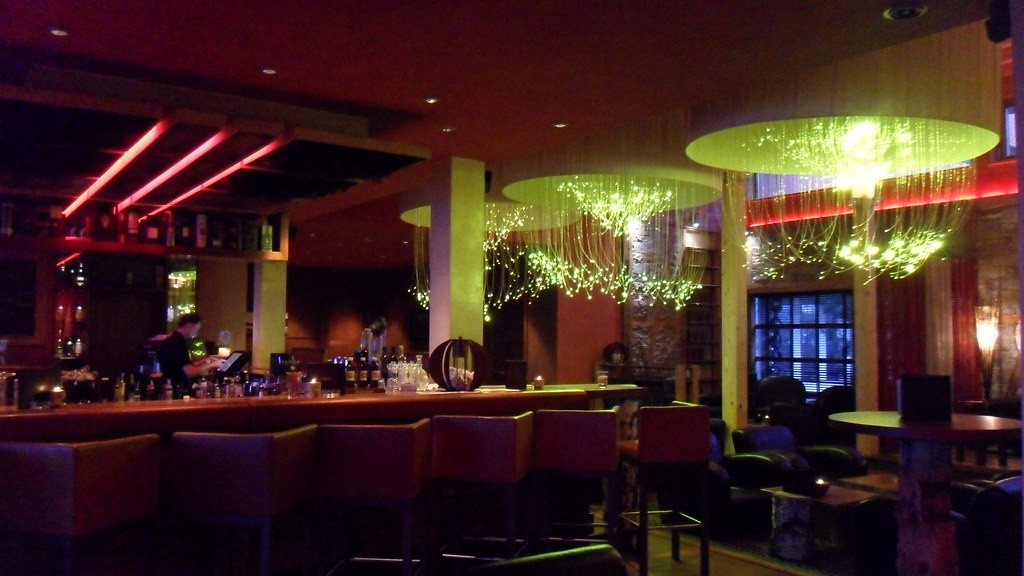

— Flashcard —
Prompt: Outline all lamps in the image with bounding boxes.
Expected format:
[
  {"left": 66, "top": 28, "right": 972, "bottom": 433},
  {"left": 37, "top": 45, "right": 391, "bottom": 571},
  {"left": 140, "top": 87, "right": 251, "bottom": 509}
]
[{"left": 401, "top": 20, "right": 1003, "bottom": 324}]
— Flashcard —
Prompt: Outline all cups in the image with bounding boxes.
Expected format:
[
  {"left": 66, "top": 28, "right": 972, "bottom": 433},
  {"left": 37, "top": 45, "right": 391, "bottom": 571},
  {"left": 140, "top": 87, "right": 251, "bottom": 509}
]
[{"left": 596, "top": 370, "right": 608, "bottom": 388}]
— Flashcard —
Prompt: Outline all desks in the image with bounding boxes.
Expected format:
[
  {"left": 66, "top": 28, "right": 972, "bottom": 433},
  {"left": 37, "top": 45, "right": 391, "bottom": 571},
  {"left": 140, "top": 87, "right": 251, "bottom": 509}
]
[
  {"left": 760, "top": 474, "right": 903, "bottom": 568},
  {"left": 830, "top": 411, "right": 1023, "bottom": 576}
]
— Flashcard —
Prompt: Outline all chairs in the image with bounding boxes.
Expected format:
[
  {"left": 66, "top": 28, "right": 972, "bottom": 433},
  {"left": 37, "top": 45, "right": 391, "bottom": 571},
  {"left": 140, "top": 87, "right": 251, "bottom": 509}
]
[{"left": 661, "top": 374, "right": 1023, "bottom": 576}]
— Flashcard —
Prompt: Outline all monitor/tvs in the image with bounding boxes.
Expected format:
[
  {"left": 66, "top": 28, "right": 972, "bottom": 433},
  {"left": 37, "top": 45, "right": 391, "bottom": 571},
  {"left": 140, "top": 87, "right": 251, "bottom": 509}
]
[{"left": 216, "top": 351, "right": 250, "bottom": 377}]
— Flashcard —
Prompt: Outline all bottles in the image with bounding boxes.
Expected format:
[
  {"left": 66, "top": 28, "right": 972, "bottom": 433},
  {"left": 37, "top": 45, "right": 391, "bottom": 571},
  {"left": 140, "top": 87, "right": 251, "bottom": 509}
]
[
  {"left": 146, "top": 214, "right": 161, "bottom": 243},
  {"left": 212, "top": 222, "right": 223, "bottom": 249},
  {"left": 96, "top": 368, "right": 284, "bottom": 403},
  {"left": 178, "top": 211, "right": 193, "bottom": 246},
  {"left": 344, "top": 344, "right": 406, "bottom": 391}
]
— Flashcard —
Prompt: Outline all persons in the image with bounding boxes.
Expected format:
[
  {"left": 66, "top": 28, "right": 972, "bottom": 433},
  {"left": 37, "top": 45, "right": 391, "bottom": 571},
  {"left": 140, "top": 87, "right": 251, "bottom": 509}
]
[
  {"left": 63, "top": 321, "right": 89, "bottom": 369},
  {"left": 158, "top": 314, "right": 227, "bottom": 389}
]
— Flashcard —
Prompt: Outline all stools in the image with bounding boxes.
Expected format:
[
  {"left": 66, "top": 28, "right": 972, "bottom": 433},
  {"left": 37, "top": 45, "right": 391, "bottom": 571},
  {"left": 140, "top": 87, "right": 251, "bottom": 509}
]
[{"left": 0, "top": 401, "right": 711, "bottom": 575}]
